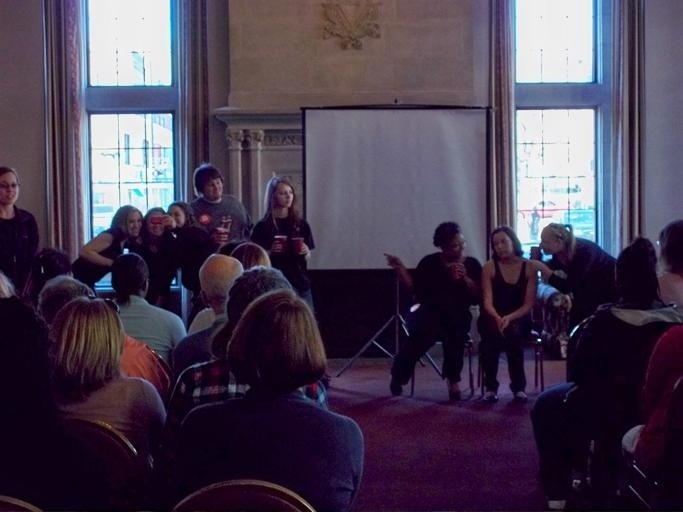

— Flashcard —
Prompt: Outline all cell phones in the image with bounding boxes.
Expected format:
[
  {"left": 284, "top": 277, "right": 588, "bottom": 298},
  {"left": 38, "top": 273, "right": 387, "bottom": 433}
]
[
  {"left": 531, "top": 247, "right": 539, "bottom": 259},
  {"left": 151, "top": 215, "right": 168, "bottom": 224}
]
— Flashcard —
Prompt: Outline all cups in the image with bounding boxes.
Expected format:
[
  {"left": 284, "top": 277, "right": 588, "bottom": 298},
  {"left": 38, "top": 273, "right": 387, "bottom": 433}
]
[
  {"left": 274, "top": 236, "right": 288, "bottom": 253},
  {"left": 216, "top": 229, "right": 230, "bottom": 243},
  {"left": 291, "top": 237, "right": 304, "bottom": 255}
]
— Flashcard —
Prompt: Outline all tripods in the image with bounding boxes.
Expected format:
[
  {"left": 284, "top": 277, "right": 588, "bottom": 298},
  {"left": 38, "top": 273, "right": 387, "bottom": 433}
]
[{"left": 336, "top": 277, "right": 444, "bottom": 396}]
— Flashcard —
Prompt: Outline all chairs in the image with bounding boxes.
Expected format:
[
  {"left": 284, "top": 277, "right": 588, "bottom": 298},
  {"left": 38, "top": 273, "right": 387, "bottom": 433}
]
[
  {"left": 563, "top": 314, "right": 595, "bottom": 385},
  {"left": 149, "top": 344, "right": 178, "bottom": 390},
  {"left": 50, "top": 412, "right": 158, "bottom": 512},
  {"left": 403, "top": 286, "right": 482, "bottom": 400},
  {"left": 168, "top": 477, "right": 320, "bottom": 512},
  {"left": 0, "top": 493, "right": 45, "bottom": 511},
  {"left": 618, "top": 371, "right": 683, "bottom": 512},
  {"left": 477, "top": 284, "right": 548, "bottom": 400}
]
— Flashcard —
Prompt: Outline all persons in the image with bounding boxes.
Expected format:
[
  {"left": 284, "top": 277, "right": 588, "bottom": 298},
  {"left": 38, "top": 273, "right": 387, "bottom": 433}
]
[
  {"left": 620, "top": 220, "right": 683, "bottom": 511},
  {"left": 481, "top": 227, "right": 539, "bottom": 403},
  {"left": 1, "top": 241, "right": 366, "bottom": 512},
  {"left": 525, "top": 223, "right": 618, "bottom": 335},
  {"left": 384, "top": 222, "right": 483, "bottom": 402},
  {"left": 1, "top": 168, "right": 40, "bottom": 297},
  {"left": 530, "top": 237, "right": 683, "bottom": 511},
  {"left": 70, "top": 164, "right": 318, "bottom": 325}
]
